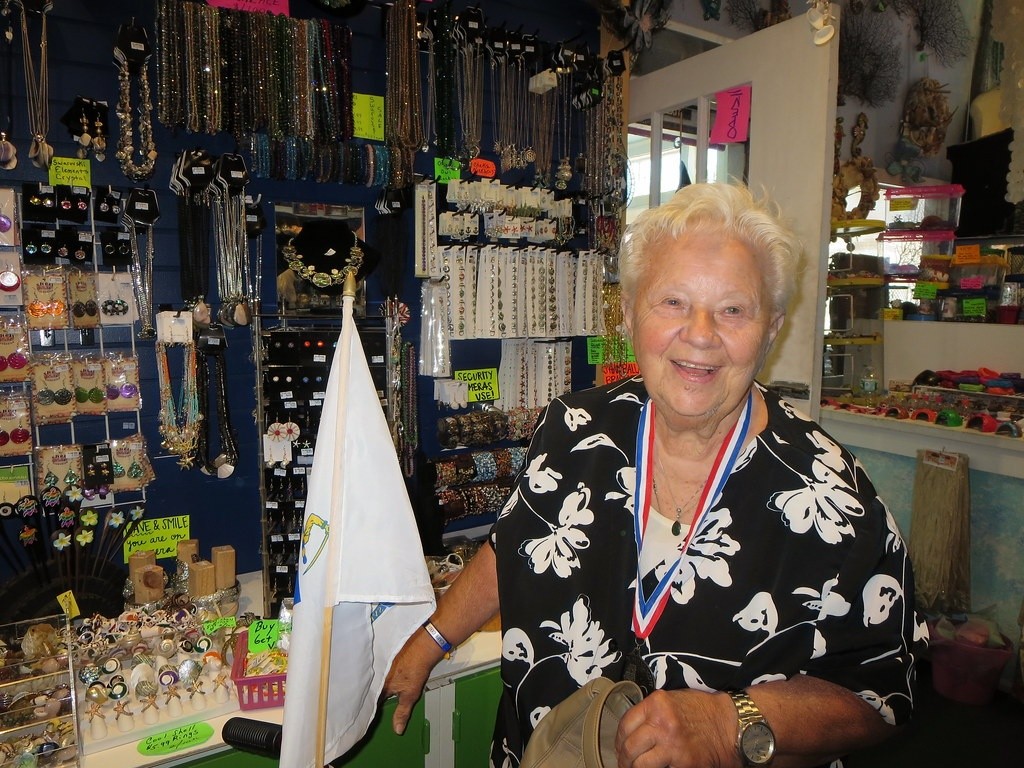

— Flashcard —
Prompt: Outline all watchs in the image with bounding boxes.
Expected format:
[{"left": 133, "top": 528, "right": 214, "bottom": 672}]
[{"left": 726, "top": 690, "right": 776, "bottom": 768}]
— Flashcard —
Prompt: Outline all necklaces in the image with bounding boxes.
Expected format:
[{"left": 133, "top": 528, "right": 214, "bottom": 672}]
[
  {"left": 384, "top": 0, "right": 605, "bottom": 199},
  {"left": 651, "top": 478, "right": 662, "bottom": 515},
  {"left": 654, "top": 440, "right": 708, "bottom": 536},
  {"left": 0, "top": 0, "right": 353, "bottom": 480}
]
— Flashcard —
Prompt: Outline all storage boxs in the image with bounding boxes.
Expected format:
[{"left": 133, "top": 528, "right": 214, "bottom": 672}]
[
  {"left": 925, "top": 618, "right": 1014, "bottom": 706},
  {"left": 230, "top": 628, "right": 287, "bottom": 712}
]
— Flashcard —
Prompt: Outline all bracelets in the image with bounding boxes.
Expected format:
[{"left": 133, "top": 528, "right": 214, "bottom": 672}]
[
  {"left": 418, "top": 192, "right": 630, "bottom": 523},
  {"left": 422, "top": 620, "right": 451, "bottom": 660}
]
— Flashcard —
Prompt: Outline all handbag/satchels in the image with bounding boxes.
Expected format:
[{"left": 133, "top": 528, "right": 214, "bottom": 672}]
[{"left": 519, "top": 676, "right": 644, "bottom": 768}]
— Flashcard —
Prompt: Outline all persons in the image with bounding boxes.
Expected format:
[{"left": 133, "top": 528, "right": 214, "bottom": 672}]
[{"left": 382, "top": 186, "right": 930, "bottom": 768}]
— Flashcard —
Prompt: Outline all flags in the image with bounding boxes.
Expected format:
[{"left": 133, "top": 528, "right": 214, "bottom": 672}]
[{"left": 279, "top": 320, "right": 437, "bottom": 768}]
